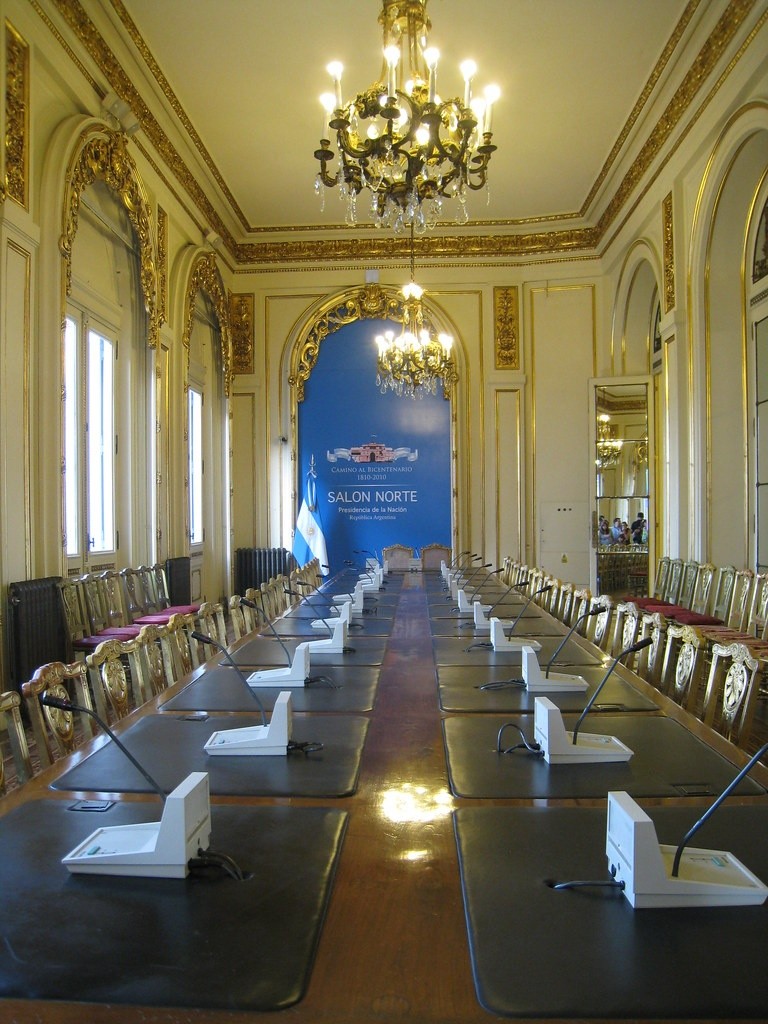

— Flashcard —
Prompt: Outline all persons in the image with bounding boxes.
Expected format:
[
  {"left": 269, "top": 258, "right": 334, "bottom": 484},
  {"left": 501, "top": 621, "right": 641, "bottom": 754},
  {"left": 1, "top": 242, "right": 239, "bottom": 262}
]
[{"left": 598, "top": 512, "right": 648, "bottom": 545}]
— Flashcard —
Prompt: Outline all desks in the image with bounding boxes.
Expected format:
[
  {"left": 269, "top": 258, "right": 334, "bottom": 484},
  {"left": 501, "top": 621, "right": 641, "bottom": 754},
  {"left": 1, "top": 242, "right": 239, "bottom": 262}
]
[{"left": 0, "top": 569, "right": 768, "bottom": 1024}]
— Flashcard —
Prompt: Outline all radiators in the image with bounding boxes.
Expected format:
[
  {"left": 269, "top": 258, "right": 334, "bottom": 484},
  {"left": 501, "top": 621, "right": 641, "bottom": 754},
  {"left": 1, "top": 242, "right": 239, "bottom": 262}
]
[
  {"left": 8, "top": 576, "right": 77, "bottom": 729},
  {"left": 165, "top": 557, "right": 192, "bottom": 607},
  {"left": 234, "top": 547, "right": 292, "bottom": 598}
]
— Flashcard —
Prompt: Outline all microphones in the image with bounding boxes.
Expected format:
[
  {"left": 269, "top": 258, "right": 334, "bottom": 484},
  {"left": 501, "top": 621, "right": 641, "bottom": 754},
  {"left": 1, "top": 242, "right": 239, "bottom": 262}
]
[
  {"left": 42, "top": 695, "right": 212, "bottom": 879},
  {"left": 189, "top": 550, "right": 384, "bottom": 755},
  {"left": 441, "top": 550, "right": 653, "bottom": 764}
]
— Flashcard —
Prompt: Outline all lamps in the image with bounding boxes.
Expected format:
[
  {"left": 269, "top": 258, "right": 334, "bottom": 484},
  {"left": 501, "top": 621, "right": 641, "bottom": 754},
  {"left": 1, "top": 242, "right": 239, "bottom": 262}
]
[
  {"left": 374, "top": 225, "right": 460, "bottom": 403},
  {"left": 314, "top": 0, "right": 504, "bottom": 235}
]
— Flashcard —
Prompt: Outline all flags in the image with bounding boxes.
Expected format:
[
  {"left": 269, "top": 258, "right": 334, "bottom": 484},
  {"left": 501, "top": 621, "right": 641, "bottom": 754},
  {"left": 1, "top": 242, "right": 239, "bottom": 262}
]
[{"left": 291, "top": 471, "right": 330, "bottom": 577}]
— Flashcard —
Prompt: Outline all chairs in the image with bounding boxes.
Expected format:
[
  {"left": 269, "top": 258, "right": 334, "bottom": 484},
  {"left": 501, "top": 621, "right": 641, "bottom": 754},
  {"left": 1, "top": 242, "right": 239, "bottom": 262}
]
[
  {"left": 500, "top": 556, "right": 763, "bottom": 747},
  {"left": 0, "top": 557, "right": 325, "bottom": 799},
  {"left": 382, "top": 544, "right": 414, "bottom": 568},
  {"left": 420, "top": 544, "right": 452, "bottom": 569},
  {"left": 598, "top": 542, "right": 768, "bottom": 662},
  {"left": 597, "top": 543, "right": 648, "bottom": 598}
]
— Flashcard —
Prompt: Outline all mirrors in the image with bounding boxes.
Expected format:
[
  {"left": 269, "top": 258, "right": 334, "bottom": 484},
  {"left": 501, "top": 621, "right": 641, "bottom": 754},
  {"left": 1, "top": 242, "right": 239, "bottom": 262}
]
[{"left": 588, "top": 376, "right": 655, "bottom": 597}]
